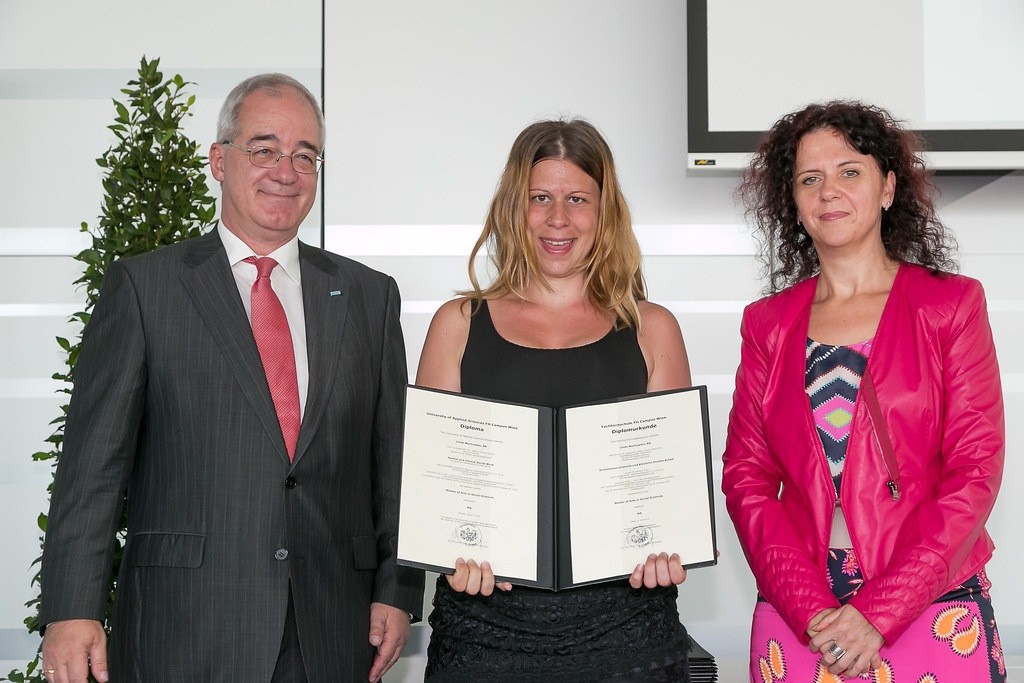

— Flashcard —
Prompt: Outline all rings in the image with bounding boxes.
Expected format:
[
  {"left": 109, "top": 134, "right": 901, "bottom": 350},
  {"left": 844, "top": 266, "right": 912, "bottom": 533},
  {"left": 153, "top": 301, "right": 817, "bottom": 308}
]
[
  {"left": 45, "top": 669, "right": 54, "bottom": 674},
  {"left": 829, "top": 644, "right": 846, "bottom": 659}
]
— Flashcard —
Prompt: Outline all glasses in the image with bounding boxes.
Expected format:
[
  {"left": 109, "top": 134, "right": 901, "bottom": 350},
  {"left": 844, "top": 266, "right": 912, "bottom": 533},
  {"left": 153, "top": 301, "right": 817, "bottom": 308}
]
[{"left": 229, "top": 141, "right": 326, "bottom": 175}]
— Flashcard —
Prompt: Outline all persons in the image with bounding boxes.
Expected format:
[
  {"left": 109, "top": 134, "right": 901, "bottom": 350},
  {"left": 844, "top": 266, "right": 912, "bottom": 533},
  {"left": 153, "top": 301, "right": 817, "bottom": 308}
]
[
  {"left": 39, "top": 75, "right": 426, "bottom": 683},
  {"left": 414, "top": 119, "right": 693, "bottom": 683},
  {"left": 720, "top": 101, "right": 1005, "bottom": 683}
]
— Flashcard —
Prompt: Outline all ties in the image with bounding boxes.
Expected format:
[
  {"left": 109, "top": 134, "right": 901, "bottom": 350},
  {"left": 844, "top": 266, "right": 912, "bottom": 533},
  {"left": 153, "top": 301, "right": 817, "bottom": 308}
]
[{"left": 239, "top": 255, "right": 301, "bottom": 464}]
